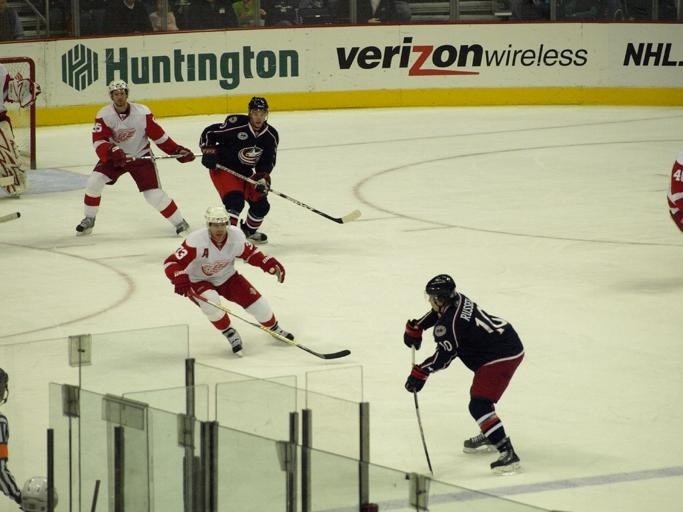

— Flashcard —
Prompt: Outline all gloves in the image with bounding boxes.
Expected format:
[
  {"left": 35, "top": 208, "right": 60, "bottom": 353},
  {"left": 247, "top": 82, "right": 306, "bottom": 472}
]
[
  {"left": 172, "top": 272, "right": 194, "bottom": 300},
  {"left": 200, "top": 149, "right": 224, "bottom": 169},
  {"left": 252, "top": 172, "right": 271, "bottom": 196},
  {"left": 261, "top": 254, "right": 285, "bottom": 282},
  {"left": 403, "top": 363, "right": 431, "bottom": 392},
  {"left": 172, "top": 145, "right": 196, "bottom": 163},
  {"left": 401, "top": 318, "right": 424, "bottom": 351},
  {"left": 97, "top": 143, "right": 127, "bottom": 173}
]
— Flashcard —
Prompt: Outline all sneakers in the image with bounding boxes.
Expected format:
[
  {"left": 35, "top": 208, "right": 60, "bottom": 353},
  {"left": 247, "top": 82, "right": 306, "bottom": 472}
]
[
  {"left": 74, "top": 215, "right": 96, "bottom": 234},
  {"left": 173, "top": 218, "right": 191, "bottom": 234}
]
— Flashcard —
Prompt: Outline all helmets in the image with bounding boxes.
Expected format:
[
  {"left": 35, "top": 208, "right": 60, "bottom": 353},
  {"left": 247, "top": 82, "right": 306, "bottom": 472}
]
[
  {"left": 0, "top": 367, "right": 8, "bottom": 409},
  {"left": 248, "top": 95, "right": 269, "bottom": 123},
  {"left": 107, "top": 79, "right": 128, "bottom": 97},
  {"left": 204, "top": 204, "right": 232, "bottom": 229},
  {"left": 423, "top": 271, "right": 457, "bottom": 308}
]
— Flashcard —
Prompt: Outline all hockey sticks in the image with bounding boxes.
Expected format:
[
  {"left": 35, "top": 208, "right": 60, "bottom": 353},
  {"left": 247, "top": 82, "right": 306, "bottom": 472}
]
[
  {"left": 191, "top": 292, "right": 351, "bottom": 359},
  {"left": 405, "top": 343, "right": 437, "bottom": 480},
  {"left": 216, "top": 165, "right": 362, "bottom": 223}
]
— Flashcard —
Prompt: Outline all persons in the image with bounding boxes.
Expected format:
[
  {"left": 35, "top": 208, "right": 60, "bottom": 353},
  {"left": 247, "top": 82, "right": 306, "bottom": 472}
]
[
  {"left": 404, "top": 274, "right": 525, "bottom": 469},
  {"left": 75, "top": 79, "right": 195, "bottom": 234},
  {"left": 666, "top": 154, "right": 683, "bottom": 231},
  {"left": 199, "top": 96, "right": 279, "bottom": 240},
  {"left": 0, "top": 367, "right": 23, "bottom": 504},
  {"left": 163, "top": 204, "right": 294, "bottom": 352},
  {"left": 508, "top": 1, "right": 678, "bottom": 19}
]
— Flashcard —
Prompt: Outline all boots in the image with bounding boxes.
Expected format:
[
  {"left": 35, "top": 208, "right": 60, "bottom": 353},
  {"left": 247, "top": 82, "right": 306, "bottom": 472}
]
[
  {"left": 221, "top": 325, "right": 243, "bottom": 355},
  {"left": 489, "top": 436, "right": 522, "bottom": 470},
  {"left": 268, "top": 322, "right": 295, "bottom": 342},
  {"left": 463, "top": 429, "right": 496, "bottom": 448},
  {"left": 240, "top": 222, "right": 267, "bottom": 242}
]
